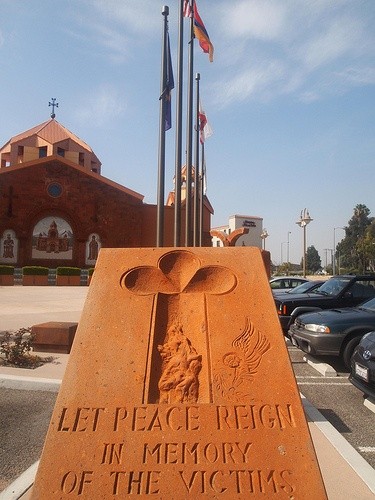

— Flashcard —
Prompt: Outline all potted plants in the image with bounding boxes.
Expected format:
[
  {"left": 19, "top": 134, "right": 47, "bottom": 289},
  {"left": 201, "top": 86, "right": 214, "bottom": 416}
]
[
  {"left": 0, "top": 266, "right": 14, "bottom": 286},
  {"left": 22, "top": 266, "right": 49, "bottom": 286},
  {"left": 55, "top": 266, "right": 81, "bottom": 285},
  {"left": 87, "top": 268, "right": 94, "bottom": 286}
]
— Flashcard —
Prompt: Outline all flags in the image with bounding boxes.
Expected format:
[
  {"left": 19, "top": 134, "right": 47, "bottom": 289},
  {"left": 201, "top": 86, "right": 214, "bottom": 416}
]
[
  {"left": 183, "top": 0, "right": 215, "bottom": 63},
  {"left": 158, "top": 31, "right": 176, "bottom": 131},
  {"left": 195, "top": 96, "right": 214, "bottom": 145}
]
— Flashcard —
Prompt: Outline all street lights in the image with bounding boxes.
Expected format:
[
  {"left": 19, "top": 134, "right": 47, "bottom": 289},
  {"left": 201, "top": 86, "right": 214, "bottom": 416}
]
[
  {"left": 260, "top": 228, "right": 269, "bottom": 251},
  {"left": 296, "top": 208, "right": 313, "bottom": 278},
  {"left": 279, "top": 227, "right": 337, "bottom": 276}
]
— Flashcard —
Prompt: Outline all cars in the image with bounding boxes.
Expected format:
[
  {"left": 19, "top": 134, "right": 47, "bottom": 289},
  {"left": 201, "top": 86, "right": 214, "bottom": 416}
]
[
  {"left": 273, "top": 281, "right": 326, "bottom": 297},
  {"left": 287, "top": 296, "right": 375, "bottom": 372},
  {"left": 269, "top": 276, "right": 310, "bottom": 296},
  {"left": 348, "top": 331, "right": 375, "bottom": 399}
]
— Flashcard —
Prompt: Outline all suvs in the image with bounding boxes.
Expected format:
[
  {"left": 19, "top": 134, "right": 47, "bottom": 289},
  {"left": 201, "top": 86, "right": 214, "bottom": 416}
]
[{"left": 273, "top": 274, "right": 375, "bottom": 342}]
